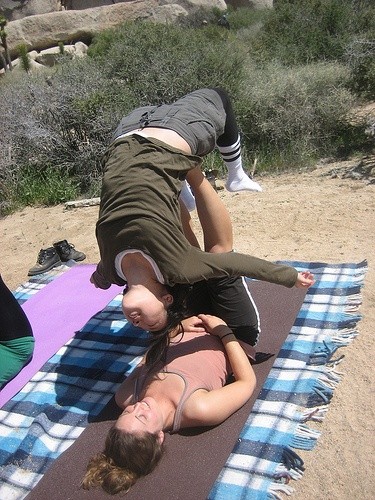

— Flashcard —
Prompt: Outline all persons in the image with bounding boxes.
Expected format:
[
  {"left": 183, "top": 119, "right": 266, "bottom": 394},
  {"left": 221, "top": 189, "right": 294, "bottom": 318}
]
[
  {"left": 78, "top": 86, "right": 314, "bottom": 494},
  {"left": 0, "top": 274, "right": 35, "bottom": 390}
]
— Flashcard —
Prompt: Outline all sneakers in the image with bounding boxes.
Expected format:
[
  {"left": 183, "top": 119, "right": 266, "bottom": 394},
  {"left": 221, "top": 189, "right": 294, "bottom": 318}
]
[
  {"left": 53, "top": 240, "right": 86, "bottom": 262},
  {"left": 28, "top": 247, "right": 62, "bottom": 276}
]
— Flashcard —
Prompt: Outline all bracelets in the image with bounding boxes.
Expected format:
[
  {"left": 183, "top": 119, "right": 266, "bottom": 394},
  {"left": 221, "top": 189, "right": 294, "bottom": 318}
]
[{"left": 220, "top": 333, "right": 233, "bottom": 341}]
